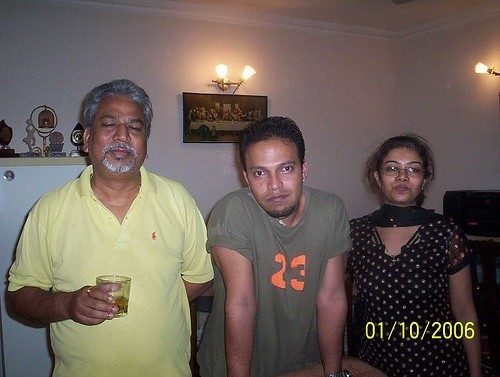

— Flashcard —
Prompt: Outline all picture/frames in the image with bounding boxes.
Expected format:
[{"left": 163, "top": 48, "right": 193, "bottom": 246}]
[{"left": 183, "top": 92, "right": 268, "bottom": 143}]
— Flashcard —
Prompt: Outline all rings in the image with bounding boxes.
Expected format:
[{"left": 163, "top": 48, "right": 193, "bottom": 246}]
[{"left": 86, "top": 285, "right": 94, "bottom": 295}]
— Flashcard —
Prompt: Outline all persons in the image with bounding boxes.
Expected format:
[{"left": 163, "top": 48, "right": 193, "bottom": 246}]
[
  {"left": 205, "top": 115, "right": 352, "bottom": 377},
  {"left": 344, "top": 134, "right": 486, "bottom": 377},
  {"left": 4, "top": 79, "right": 215, "bottom": 377}
]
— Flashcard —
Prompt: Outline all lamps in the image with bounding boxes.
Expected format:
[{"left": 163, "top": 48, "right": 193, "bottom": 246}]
[
  {"left": 474, "top": 61, "right": 500, "bottom": 76},
  {"left": 212, "top": 64, "right": 257, "bottom": 95}
]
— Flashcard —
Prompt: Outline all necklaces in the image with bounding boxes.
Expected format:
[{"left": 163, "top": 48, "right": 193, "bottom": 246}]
[
  {"left": 380, "top": 226, "right": 423, "bottom": 265},
  {"left": 271, "top": 211, "right": 297, "bottom": 228}
]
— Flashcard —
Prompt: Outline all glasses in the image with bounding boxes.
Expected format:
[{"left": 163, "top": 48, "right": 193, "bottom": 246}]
[{"left": 376, "top": 166, "right": 427, "bottom": 178}]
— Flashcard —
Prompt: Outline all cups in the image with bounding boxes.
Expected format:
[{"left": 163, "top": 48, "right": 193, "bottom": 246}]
[{"left": 96, "top": 275, "right": 131, "bottom": 318}]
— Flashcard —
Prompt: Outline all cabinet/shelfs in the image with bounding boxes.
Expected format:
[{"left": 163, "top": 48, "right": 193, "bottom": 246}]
[{"left": 0, "top": 155, "right": 94, "bottom": 377}]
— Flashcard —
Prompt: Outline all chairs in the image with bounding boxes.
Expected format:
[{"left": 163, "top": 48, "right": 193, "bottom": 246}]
[{"left": 443, "top": 191, "right": 500, "bottom": 307}]
